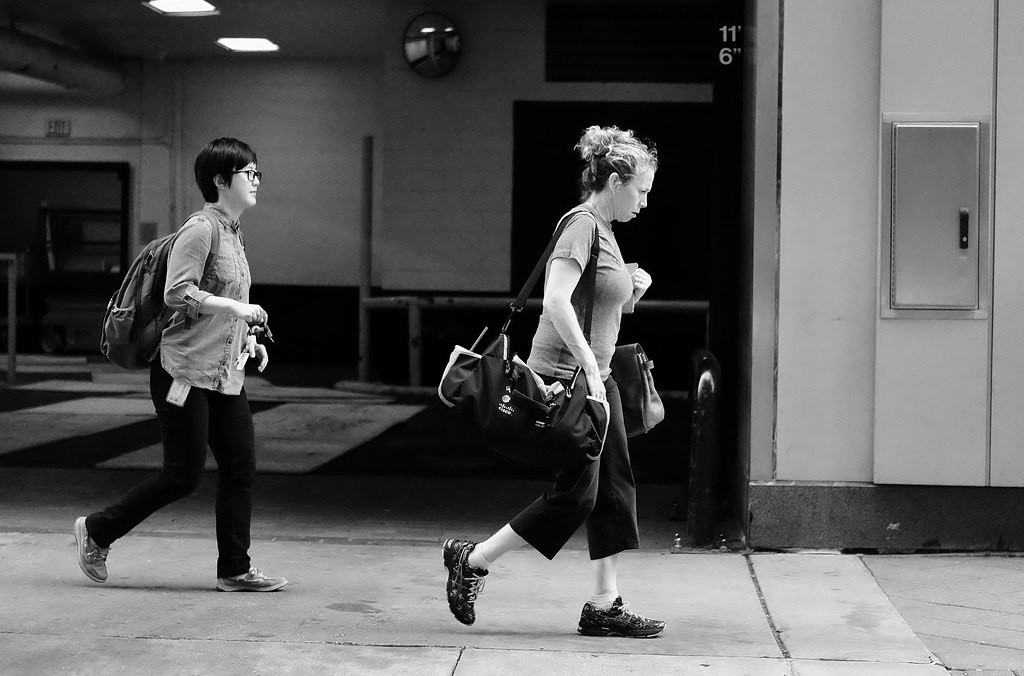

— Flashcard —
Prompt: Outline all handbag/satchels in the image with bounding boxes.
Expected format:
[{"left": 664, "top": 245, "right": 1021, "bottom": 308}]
[
  {"left": 609, "top": 343, "right": 665, "bottom": 439},
  {"left": 437, "top": 334, "right": 610, "bottom": 462}
]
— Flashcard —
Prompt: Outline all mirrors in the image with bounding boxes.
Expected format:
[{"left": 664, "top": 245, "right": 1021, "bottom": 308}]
[{"left": 402, "top": 12, "right": 462, "bottom": 78}]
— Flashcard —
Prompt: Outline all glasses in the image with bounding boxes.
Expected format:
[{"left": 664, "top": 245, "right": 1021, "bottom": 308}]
[{"left": 232, "top": 170, "right": 262, "bottom": 181}]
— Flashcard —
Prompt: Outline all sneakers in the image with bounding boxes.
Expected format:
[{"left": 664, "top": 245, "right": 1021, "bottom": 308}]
[
  {"left": 442, "top": 539, "right": 489, "bottom": 626},
  {"left": 74, "top": 516, "right": 112, "bottom": 583},
  {"left": 577, "top": 596, "right": 665, "bottom": 637},
  {"left": 216, "top": 569, "right": 288, "bottom": 592}
]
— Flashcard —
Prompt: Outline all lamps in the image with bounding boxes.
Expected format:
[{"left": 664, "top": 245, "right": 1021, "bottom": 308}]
[
  {"left": 217, "top": 38, "right": 279, "bottom": 52},
  {"left": 150, "top": 0, "right": 215, "bottom": 13}
]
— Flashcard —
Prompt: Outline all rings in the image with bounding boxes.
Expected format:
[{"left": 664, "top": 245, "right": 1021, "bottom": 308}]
[{"left": 639, "top": 278, "right": 643, "bottom": 284}]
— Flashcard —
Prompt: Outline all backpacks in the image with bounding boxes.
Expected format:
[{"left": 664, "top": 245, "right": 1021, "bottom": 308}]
[{"left": 100, "top": 211, "right": 244, "bottom": 370}]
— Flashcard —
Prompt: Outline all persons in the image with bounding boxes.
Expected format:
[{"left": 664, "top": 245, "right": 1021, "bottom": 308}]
[
  {"left": 442, "top": 125, "right": 666, "bottom": 639},
  {"left": 74, "top": 137, "right": 289, "bottom": 592}
]
